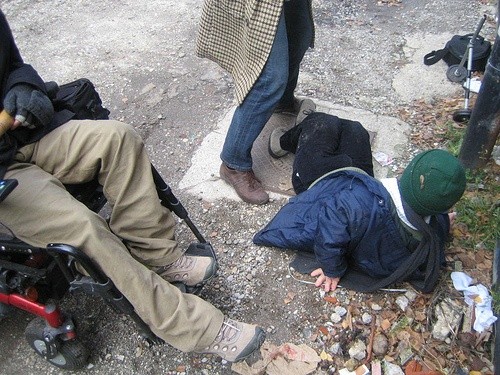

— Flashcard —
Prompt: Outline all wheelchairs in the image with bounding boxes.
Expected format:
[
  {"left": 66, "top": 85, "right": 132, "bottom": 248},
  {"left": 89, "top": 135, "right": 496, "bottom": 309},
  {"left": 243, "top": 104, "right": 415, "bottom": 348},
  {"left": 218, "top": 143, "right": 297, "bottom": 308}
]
[{"left": 0, "top": 78, "right": 219, "bottom": 370}]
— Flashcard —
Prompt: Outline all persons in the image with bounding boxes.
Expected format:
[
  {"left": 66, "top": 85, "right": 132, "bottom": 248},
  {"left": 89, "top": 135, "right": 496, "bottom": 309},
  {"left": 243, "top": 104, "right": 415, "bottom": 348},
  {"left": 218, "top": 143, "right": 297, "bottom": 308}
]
[
  {"left": 196, "top": 0, "right": 315, "bottom": 204},
  {"left": 254, "top": 98, "right": 467, "bottom": 292},
  {"left": 0, "top": 8, "right": 265, "bottom": 362}
]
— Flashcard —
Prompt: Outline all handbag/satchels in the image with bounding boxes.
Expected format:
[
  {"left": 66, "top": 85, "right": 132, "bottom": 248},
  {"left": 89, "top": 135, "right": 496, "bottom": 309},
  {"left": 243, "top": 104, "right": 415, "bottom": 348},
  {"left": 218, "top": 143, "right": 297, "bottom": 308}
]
[{"left": 424, "top": 33, "right": 491, "bottom": 73}]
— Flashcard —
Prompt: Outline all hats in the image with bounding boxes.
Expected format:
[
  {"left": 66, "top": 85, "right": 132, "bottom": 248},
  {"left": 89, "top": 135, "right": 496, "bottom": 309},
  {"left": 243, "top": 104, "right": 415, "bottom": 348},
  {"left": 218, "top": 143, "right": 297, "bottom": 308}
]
[{"left": 401, "top": 148, "right": 467, "bottom": 217}]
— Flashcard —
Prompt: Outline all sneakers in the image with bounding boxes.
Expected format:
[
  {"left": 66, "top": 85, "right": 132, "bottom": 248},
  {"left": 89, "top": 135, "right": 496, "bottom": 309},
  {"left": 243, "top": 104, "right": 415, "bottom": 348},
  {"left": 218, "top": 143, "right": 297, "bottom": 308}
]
[
  {"left": 193, "top": 314, "right": 266, "bottom": 362},
  {"left": 147, "top": 254, "right": 216, "bottom": 286},
  {"left": 273, "top": 96, "right": 302, "bottom": 113},
  {"left": 219, "top": 162, "right": 269, "bottom": 205}
]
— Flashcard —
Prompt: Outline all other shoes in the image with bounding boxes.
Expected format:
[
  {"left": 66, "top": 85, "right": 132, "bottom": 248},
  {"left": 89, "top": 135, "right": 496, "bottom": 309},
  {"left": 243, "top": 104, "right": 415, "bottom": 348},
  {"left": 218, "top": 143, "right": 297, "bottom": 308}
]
[
  {"left": 296, "top": 98, "right": 316, "bottom": 128},
  {"left": 267, "top": 127, "right": 291, "bottom": 159}
]
